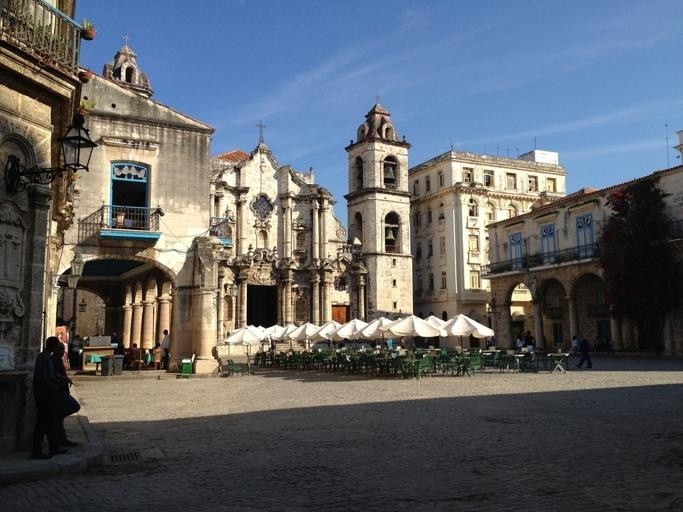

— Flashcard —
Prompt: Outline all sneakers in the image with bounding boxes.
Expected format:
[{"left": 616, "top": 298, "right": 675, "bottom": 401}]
[{"left": 61, "top": 438, "right": 78, "bottom": 448}]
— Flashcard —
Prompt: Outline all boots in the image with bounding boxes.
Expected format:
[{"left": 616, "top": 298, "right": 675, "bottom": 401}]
[
  {"left": 29, "top": 446, "right": 52, "bottom": 460},
  {"left": 49, "top": 441, "right": 69, "bottom": 456}
]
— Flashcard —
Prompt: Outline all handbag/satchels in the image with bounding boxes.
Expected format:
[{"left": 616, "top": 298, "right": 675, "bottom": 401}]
[{"left": 64, "top": 396, "right": 80, "bottom": 417}]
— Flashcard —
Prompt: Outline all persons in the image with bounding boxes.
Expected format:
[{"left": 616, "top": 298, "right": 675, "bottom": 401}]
[
  {"left": 29, "top": 336, "right": 68, "bottom": 460},
  {"left": 56, "top": 316, "right": 86, "bottom": 370},
  {"left": 45, "top": 342, "right": 83, "bottom": 447},
  {"left": 109, "top": 328, "right": 170, "bottom": 372},
  {"left": 516, "top": 330, "right": 611, "bottom": 369}
]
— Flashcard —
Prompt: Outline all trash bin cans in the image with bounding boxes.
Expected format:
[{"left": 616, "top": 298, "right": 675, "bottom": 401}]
[
  {"left": 181, "top": 358, "right": 192, "bottom": 378},
  {"left": 100, "top": 355, "right": 124, "bottom": 376}
]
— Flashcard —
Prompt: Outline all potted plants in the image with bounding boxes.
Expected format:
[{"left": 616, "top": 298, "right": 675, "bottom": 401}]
[{"left": 77, "top": 16, "right": 97, "bottom": 120}]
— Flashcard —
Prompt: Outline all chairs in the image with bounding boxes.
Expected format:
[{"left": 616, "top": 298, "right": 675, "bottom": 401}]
[{"left": 227, "top": 337, "right": 574, "bottom": 379}]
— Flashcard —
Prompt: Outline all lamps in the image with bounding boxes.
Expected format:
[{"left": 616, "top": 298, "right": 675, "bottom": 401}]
[
  {"left": 3, "top": 116, "right": 99, "bottom": 196},
  {"left": 50, "top": 244, "right": 88, "bottom": 312}
]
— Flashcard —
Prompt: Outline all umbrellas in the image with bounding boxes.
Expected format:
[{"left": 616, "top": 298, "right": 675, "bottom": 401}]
[{"left": 223, "top": 313, "right": 496, "bottom": 357}]
